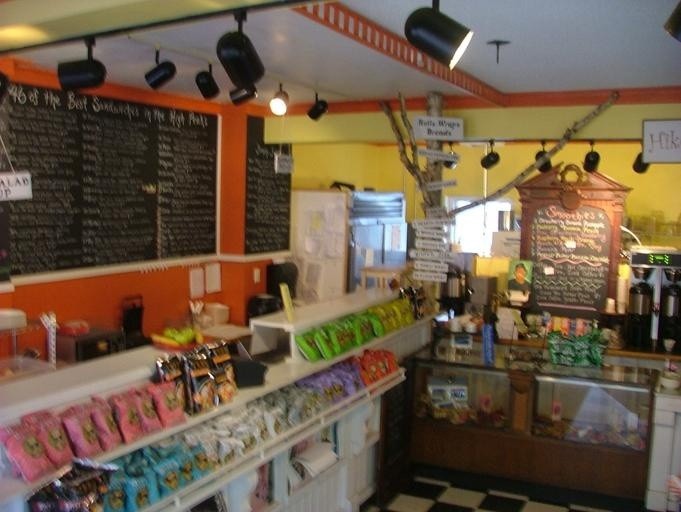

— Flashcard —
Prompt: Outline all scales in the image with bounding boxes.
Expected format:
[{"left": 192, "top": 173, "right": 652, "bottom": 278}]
[{"left": 630, "top": 246, "right": 681, "bottom": 351}]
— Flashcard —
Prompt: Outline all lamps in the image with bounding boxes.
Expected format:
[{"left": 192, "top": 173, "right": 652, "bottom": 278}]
[
  {"left": 53, "top": 12, "right": 333, "bottom": 125},
  {"left": 442, "top": 138, "right": 651, "bottom": 177},
  {"left": 400, "top": 0, "right": 476, "bottom": 74}
]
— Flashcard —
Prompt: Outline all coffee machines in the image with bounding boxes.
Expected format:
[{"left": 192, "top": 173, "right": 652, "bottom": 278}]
[{"left": 624, "top": 243, "right": 681, "bottom": 352}]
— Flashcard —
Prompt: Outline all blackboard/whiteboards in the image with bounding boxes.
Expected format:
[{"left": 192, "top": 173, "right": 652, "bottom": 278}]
[
  {"left": 244, "top": 115, "right": 292, "bottom": 254},
  {"left": 525, "top": 198, "right": 618, "bottom": 314},
  {"left": 0, "top": 76, "right": 223, "bottom": 286}
]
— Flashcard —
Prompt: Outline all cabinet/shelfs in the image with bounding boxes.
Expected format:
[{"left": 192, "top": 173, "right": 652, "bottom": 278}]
[
  {"left": 1, "top": 289, "right": 436, "bottom": 512},
  {"left": 404, "top": 336, "right": 661, "bottom": 508}
]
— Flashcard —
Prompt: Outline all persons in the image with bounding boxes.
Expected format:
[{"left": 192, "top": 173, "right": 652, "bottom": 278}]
[{"left": 509, "top": 263, "right": 531, "bottom": 295}]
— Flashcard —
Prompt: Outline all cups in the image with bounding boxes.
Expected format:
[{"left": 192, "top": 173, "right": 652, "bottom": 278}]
[{"left": 603, "top": 275, "right": 629, "bottom": 316}]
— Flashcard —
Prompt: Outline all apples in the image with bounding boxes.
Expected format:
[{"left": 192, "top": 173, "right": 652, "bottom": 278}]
[{"left": 163, "top": 326, "right": 194, "bottom": 344}]
